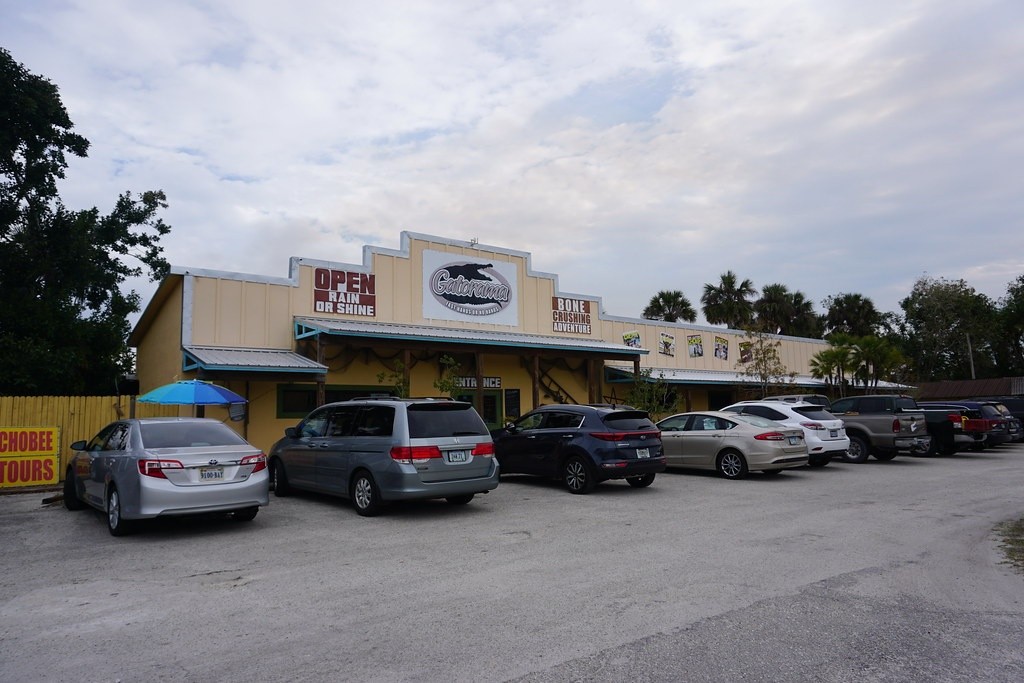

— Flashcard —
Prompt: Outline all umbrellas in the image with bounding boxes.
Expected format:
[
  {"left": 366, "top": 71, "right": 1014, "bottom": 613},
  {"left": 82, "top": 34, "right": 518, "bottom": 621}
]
[{"left": 134, "top": 380, "right": 249, "bottom": 418}]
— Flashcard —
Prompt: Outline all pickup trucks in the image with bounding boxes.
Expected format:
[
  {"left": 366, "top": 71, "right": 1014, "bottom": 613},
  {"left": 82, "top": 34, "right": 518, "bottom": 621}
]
[{"left": 893, "top": 405, "right": 989, "bottom": 454}]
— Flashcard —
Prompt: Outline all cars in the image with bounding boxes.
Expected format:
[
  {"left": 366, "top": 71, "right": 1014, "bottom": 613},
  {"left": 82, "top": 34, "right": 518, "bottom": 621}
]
[
  {"left": 62, "top": 416, "right": 271, "bottom": 536},
  {"left": 914, "top": 400, "right": 1010, "bottom": 447},
  {"left": 652, "top": 411, "right": 809, "bottom": 479},
  {"left": 488, "top": 402, "right": 669, "bottom": 495},
  {"left": 976, "top": 401, "right": 1024, "bottom": 441}
]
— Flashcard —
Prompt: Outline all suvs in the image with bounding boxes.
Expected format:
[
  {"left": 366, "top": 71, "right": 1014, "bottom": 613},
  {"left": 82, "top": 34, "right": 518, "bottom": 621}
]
[
  {"left": 719, "top": 400, "right": 850, "bottom": 470},
  {"left": 819, "top": 394, "right": 931, "bottom": 463}
]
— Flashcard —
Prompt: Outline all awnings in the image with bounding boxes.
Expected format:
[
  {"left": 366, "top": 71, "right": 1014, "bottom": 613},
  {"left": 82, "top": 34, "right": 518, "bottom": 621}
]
[
  {"left": 293, "top": 314, "right": 649, "bottom": 354},
  {"left": 605, "top": 363, "right": 916, "bottom": 391},
  {"left": 182, "top": 343, "right": 329, "bottom": 374}
]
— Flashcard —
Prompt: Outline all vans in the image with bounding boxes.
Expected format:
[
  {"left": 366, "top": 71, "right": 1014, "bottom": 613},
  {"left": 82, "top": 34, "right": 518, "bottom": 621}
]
[
  {"left": 265, "top": 395, "right": 501, "bottom": 519},
  {"left": 760, "top": 393, "right": 834, "bottom": 413}
]
